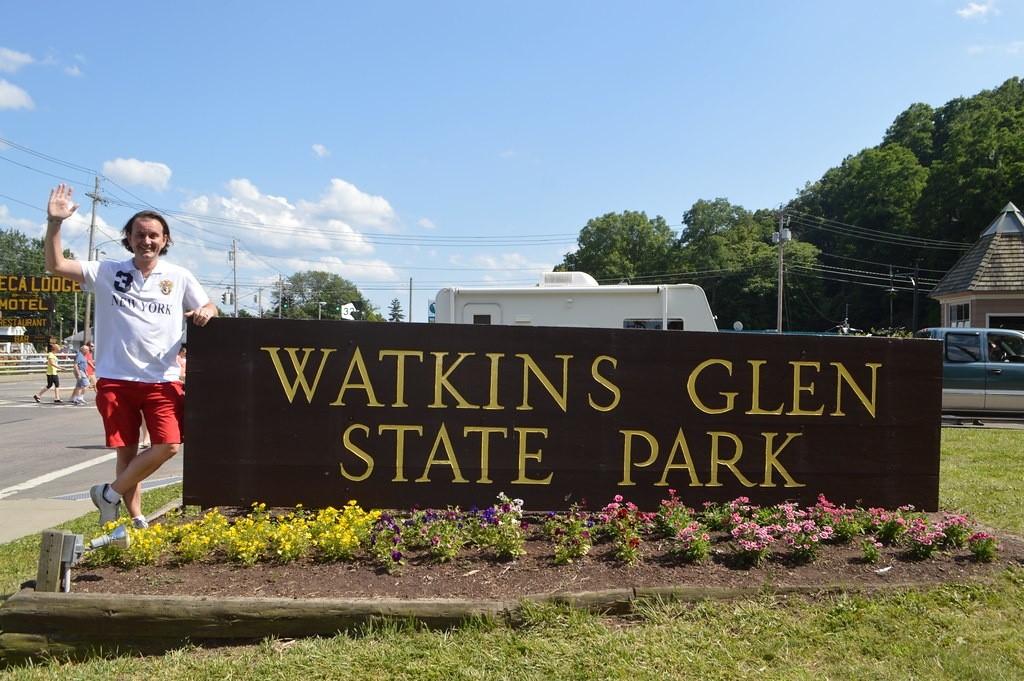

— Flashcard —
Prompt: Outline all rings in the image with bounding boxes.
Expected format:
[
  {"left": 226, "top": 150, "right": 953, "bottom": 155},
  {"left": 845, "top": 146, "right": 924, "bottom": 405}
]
[{"left": 205, "top": 313, "right": 208, "bottom": 317}]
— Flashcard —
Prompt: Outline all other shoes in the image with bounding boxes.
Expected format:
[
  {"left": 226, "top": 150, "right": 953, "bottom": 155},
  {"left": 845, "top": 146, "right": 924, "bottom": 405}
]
[
  {"left": 53, "top": 398, "right": 63, "bottom": 403},
  {"left": 67, "top": 397, "right": 86, "bottom": 406},
  {"left": 955, "top": 419, "right": 963, "bottom": 424},
  {"left": 973, "top": 420, "right": 984, "bottom": 425},
  {"left": 33, "top": 395, "right": 41, "bottom": 402}
]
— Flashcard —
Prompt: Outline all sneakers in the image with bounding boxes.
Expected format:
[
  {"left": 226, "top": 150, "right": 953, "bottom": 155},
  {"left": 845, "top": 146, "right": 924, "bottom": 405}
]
[
  {"left": 130, "top": 515, "right": 150, "bottom": 532},
  {"left": 89, "top": 483, "right": 121, "bottom": 531}
]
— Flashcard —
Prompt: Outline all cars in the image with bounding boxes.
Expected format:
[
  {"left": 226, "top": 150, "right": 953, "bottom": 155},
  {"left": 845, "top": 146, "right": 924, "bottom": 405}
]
[{"left": 57, "top": 349, "right": 78, "bottom": 364}]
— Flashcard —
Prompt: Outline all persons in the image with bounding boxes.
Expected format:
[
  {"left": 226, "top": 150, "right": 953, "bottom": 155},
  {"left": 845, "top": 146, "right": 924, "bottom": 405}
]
[
  {"left": 68, "top": 342, "right": 99, "bottom": 403},
  {"left": 177, "top": 348, "right": 187, "bottom": 385},
  {"left": 955, "top": 419, "right": 984, "bottom": 425},
  {"left": 44, "top": 183, "right": 218, "bottom": 529},
  {"left": 34, "top": 344, "right": 64, "bottom": 402},
  {"left": 74, "top": 345, "right": 90, "bottom": 404}
]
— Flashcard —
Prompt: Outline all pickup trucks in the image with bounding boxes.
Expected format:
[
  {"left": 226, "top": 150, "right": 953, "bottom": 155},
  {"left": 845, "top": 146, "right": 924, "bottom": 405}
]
[{"left": 907, "top": 325, "right": 1024, "bottom": 424}]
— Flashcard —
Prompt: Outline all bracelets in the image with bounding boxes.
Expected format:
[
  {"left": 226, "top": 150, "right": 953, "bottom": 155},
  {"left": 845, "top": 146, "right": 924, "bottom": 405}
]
[{"left": 47, "top": 218, "right": 62, "bottom": 224}]
